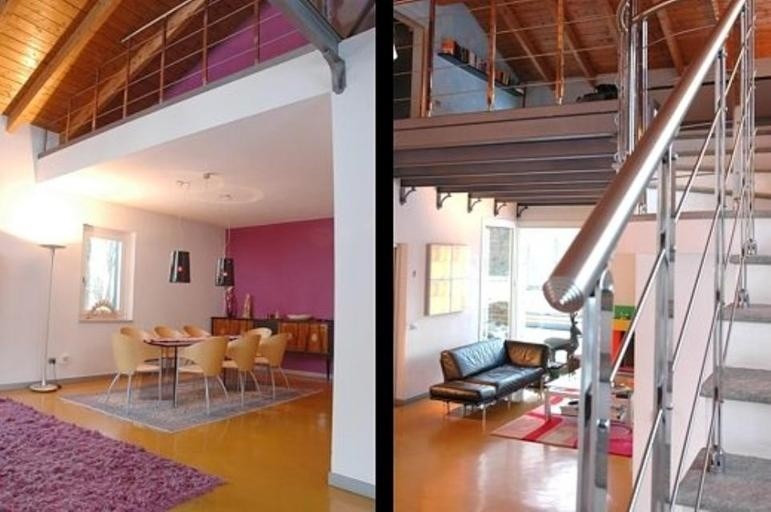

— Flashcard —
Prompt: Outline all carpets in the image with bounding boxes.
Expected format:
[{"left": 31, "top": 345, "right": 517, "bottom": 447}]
[
  {"left": 489, "top": 393, "right": 633, "bottom": 458},
  {"left": 55, "top": 375, "right": 326, "bottom": 434},
  {"left": 1, "top": 392, "right": 233, "bottom": 512}
]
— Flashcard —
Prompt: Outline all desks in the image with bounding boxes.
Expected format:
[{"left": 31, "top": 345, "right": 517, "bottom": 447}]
[{"left": 209, "top": 315, "right": 334, "bottom": 391}]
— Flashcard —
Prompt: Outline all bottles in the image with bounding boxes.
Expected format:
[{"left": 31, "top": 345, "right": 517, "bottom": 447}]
[{"left": 243, "top": 292, "right": 254, "bottom": 320}]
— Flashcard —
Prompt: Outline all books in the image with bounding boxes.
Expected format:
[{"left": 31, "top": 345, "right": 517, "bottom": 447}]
[{"left": 441, "top": 36, "right": 525, "bottom": 96}]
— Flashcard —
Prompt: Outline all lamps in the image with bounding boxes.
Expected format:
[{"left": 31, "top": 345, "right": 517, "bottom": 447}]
[
  {"left": 26, "top": 241, "right": 67, "bottom": 392},
  {"left": 216, "top": 194, "right": 236, "bottom": 287},
  {"left": 168, "top": 180, "right": 192, "bottom": 286}
]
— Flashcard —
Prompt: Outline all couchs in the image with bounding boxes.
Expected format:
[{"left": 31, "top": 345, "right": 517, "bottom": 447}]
[{"left": 430, "top": 337, "right": 551, "bottom": 436}]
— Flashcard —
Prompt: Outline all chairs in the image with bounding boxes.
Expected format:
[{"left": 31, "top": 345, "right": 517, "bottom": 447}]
[{"left": 103, "top": 322, "right": 292, "bottom": 417}]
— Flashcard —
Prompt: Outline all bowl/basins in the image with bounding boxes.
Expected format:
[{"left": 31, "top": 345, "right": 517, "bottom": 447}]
[{"left": 288, "top": 314, "right": 312, "bottom": 321}]
